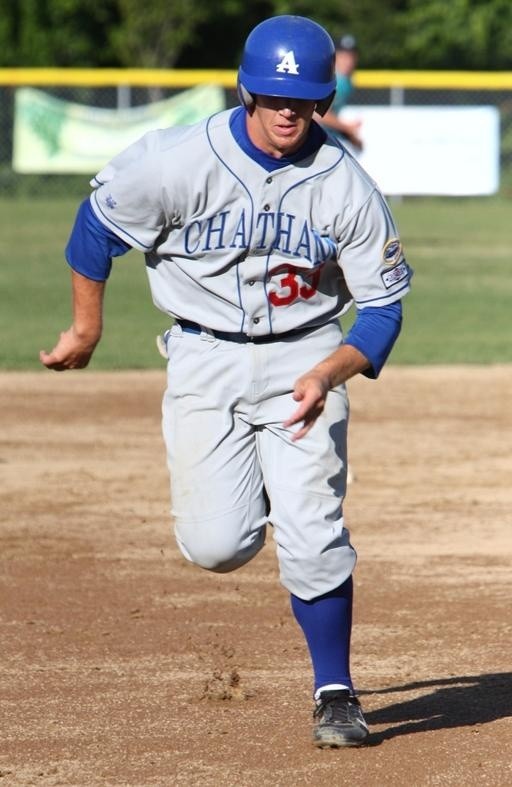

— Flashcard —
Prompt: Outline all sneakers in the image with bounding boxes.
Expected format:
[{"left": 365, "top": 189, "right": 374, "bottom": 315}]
[{"left": 312, "top": 684, "right": 369, "bottom": 747}]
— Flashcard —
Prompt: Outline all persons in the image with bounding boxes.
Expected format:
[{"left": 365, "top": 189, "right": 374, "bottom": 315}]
[
  {"left": 37, "top": 14, "right": 417, "bottom": 747},
  {"left": 310, "top": 33, "right": 363, "bottom": 160}
]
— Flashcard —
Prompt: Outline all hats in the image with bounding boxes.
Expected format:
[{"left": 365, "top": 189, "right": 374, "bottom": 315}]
[
  {"left": 237, "top": 16, "right": 337, "bottom": 117},
  {"left": 335, "top": 34, "right": 362, "bottom": 53}
]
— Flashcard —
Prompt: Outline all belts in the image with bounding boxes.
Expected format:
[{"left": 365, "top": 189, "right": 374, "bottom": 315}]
[{"left": 174, "top": 317, "right": 321, "bottom": 344}]
